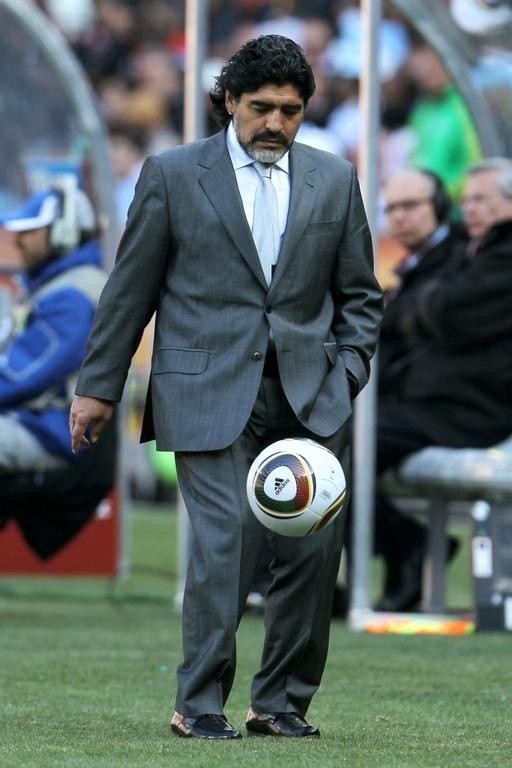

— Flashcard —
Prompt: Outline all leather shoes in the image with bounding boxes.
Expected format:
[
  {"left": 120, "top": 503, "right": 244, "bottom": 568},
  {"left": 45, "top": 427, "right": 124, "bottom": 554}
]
[
  {"left": 372, "top": 534, "right": 458, "bottom": 610},
  {"left": 246, "top": 704, "right": 320, "bottom": 737},
  {"left": 170, "top": 711, "right": 242, "bottom": 739}
]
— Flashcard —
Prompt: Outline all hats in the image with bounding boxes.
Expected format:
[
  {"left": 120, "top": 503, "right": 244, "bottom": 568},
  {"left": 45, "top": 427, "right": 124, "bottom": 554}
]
[{"left": 0, "top": 186, "right": 97, "bottom": 232}]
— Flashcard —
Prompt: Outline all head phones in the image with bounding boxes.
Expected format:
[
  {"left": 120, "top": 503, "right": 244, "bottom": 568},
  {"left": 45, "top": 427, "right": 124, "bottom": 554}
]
[
  {"left": 422, "top": 170, "right": 452, "bottom": 223},
  {"left": 49, "top": 172, "right": 82, "bottom": 250}
]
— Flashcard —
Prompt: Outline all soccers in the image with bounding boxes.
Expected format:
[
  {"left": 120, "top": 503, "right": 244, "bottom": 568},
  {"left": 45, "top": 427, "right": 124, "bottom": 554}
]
[{"left": 246, "top": 438, "right": 348, "bottom": 537}]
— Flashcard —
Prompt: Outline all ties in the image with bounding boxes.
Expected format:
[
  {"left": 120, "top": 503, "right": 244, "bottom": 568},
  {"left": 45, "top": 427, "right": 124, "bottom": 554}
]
[{"left": 252, "top": 161, "right": 281, "bottom": 288}]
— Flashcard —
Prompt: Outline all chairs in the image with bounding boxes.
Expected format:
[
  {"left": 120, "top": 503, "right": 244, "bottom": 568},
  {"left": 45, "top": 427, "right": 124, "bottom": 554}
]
[{"left": 398, "top": 428, "right": 510, "bottom": 614}]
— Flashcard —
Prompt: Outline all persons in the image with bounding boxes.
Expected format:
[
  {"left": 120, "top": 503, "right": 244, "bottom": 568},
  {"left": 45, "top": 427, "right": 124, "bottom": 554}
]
[
  {"left": 66, "top": 35, "right": 385, "bottom": 737},
  {"left": 0, "top": 0, "right": 512, "bottom": 616}
]
